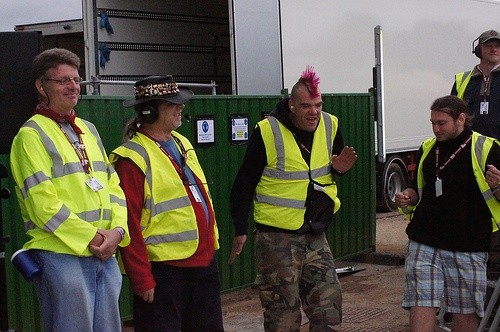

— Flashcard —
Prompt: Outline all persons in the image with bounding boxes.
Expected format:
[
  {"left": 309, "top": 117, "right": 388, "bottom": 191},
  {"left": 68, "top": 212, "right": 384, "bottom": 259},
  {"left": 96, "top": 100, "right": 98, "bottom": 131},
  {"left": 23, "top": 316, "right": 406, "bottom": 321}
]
[
  {"left": 10, "top": 48, "right": 131, "bottom": 332},
  {"left": 395, "top": 94, "right": 500, "bottom": 332},
  {"left": 228, "top": 65, "right": 357, "bottom": 332},
  {"left": 109, "top": 73, "right": 225, "bottom": 332},
  {"left": 449, "top": 30, "right": 500, "bottom": 143}
]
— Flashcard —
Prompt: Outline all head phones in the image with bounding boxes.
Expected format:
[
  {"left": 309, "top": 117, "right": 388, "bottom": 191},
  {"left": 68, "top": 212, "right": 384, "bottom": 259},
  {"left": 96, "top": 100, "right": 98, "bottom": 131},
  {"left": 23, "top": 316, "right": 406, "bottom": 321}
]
[{"left": 472, "top": 34, "right": 481, "bottom": 57}]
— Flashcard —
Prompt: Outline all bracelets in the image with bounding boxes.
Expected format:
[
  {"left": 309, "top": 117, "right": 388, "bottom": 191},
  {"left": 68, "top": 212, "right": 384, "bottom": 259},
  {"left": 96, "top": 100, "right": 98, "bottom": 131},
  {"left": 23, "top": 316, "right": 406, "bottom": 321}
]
[
  {"left": 491, "top": 182, "right": 500, "bottom": 193},
  {"left": 114, "top": 228, "right": 124, "bottom": 240}
]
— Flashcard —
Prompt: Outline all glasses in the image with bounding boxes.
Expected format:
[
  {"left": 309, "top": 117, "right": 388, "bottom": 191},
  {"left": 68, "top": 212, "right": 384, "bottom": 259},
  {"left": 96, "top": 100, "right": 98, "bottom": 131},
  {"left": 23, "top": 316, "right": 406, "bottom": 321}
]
[{"left": 44, "top": 76, "right": 83, "bottom": 85}]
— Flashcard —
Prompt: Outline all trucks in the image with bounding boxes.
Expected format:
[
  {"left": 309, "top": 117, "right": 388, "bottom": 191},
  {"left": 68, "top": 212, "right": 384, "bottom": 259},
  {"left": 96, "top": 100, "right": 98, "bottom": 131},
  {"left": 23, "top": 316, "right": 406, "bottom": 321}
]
[{"left": 83, "top": 0, "right": 500, "bottom": 212}]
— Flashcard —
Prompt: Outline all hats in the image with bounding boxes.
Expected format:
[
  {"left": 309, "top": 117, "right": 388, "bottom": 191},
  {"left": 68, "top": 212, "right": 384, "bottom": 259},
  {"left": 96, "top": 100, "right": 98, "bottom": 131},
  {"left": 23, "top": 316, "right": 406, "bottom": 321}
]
[
  {"left": 478, "top": 29, "right": 500, "bottom": 45},
  {"left": 123, "top": 75, "right": 194, "bottom": 107}
]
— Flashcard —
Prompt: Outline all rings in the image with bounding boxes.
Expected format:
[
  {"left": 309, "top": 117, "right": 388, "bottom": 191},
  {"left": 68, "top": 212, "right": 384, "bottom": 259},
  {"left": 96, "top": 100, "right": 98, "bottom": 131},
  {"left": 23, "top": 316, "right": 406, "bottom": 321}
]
[{"left": 99, "top": 254, "right": 103, "bottom": 256}]
